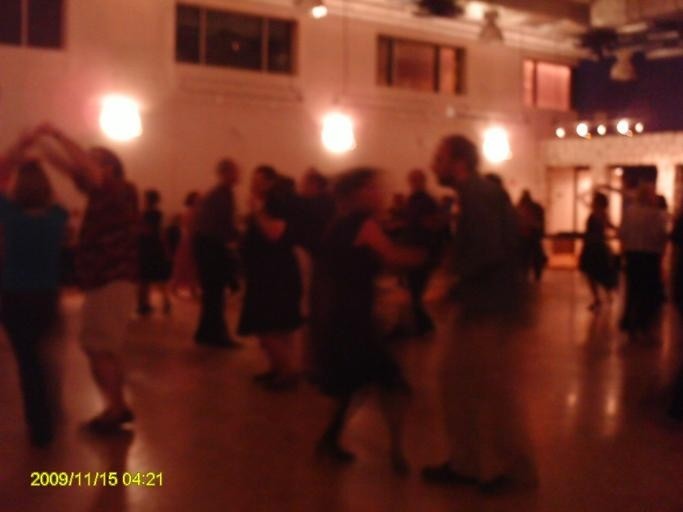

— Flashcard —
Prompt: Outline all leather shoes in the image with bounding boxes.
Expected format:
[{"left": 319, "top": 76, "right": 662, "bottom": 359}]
[
  {"left": 193, "top": 332, "right": 244, "bottom": 349},
  {"left": 420, "top": 463, "right": 538, "bottom": 496}
]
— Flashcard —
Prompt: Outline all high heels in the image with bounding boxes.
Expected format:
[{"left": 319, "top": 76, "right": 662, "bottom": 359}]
[
  {"left": 315, "top": 440, "right": 411, "bottom": 476},
  {"left": 91, "top": 407, "right": 135, "bottom": 429},
  {"left": 254, "top": 370, "right": 299, "bottom": 392}
]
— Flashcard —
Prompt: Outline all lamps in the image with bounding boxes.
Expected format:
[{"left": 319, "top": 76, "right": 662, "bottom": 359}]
[
  {"left": 479, "top": 10, "right": 502, "bottom": 42},
  {"left": 309, "top": 0, "right": 330, "bottom": 18}
]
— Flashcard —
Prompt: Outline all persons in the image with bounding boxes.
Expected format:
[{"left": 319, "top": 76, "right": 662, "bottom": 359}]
[{"left": 1, "top": 119, "right": 683, "bottom": 496}]
